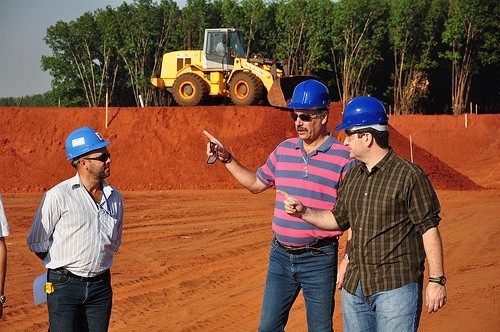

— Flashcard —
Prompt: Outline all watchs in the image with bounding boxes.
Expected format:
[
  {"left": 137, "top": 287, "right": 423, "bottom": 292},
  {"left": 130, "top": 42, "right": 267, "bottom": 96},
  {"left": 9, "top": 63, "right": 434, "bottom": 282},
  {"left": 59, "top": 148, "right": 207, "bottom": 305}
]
[
  {"left": 0, "top": 295, "right": 6, "bottom": 303},
  {"left": 429, "top": 276, "right": 446, "bottom": 286}
]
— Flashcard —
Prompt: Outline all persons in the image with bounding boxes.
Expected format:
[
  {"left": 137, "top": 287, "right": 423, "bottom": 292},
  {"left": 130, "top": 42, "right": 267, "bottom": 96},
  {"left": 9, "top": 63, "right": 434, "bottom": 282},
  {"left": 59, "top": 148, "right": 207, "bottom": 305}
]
[
  {"left": 275, "top": 95, "right": 447, "bottom": 332},
  {"left": 203, "top": 79, "right": 352, "bottom": 332},
  {"left": 27, "top": 126, "right": 124, "bottom": 332}
]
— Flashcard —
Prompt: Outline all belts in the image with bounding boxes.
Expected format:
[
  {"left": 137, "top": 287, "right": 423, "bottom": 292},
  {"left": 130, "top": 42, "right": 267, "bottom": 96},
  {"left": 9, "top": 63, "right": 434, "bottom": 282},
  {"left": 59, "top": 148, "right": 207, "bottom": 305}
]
[
  {"left": 48, "top": 268, "right": 110, "bottom": 280},
  {"left": 274, "top": 237, "right": 337, "bottom": 255}
]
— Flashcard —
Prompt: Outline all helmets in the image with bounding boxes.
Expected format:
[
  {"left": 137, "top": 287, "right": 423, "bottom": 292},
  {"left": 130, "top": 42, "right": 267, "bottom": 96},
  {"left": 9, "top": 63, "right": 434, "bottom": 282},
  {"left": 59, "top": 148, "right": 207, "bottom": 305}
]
[
  {"left": 66, "top": 126, "right": 111, "bottom": 161},
  {"left": 287, "top": 79, "right": 329, "bottom": 109},
  {"left": 335, "top": 95, "right": 389, "bottom": 130}
]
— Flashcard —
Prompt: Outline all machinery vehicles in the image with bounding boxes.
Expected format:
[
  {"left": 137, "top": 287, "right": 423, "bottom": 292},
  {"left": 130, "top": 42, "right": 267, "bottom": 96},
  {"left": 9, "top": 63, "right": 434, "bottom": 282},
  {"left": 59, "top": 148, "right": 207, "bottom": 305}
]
[{"left": 150, "top": 27, "right": 319, "bottom": 109}]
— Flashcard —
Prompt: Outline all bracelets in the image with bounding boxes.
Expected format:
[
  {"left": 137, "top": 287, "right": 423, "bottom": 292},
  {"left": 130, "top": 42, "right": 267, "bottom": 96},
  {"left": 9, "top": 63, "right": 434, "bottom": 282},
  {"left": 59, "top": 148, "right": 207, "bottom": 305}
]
[
  {"left": 221, "top": 152, "right": 231, "bottom": 163},
  {"left": 343, "top": 255, "right": 350, "bottom": 259}
]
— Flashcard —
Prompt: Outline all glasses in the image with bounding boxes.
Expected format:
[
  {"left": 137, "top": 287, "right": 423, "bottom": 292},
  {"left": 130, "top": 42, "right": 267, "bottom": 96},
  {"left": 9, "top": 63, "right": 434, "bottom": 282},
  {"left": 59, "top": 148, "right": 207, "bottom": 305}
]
[
  {"left": 345, "top": 130, "right": 363, "bottom": 136},
  {"left": 77, "top": 153, "right": 110, "bottom": 164},
  {"left": 291, "top": 113, "right": 318, "bottom": 121},
  {"left": 301, "top": 155, "right": 309, "bottom": 178},
  {"left": 207, "top": 142, "right": 218, "bottom": 164}
]
